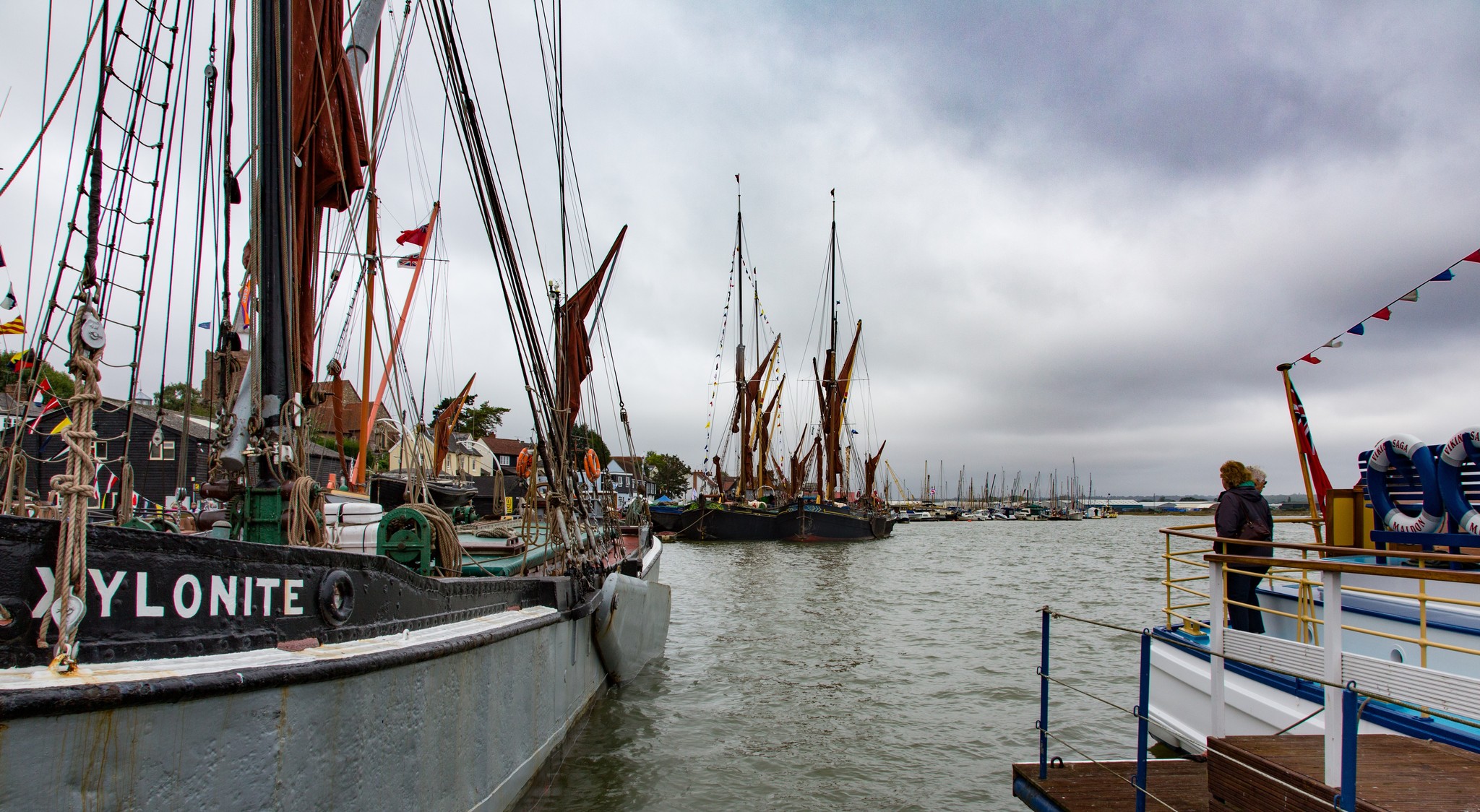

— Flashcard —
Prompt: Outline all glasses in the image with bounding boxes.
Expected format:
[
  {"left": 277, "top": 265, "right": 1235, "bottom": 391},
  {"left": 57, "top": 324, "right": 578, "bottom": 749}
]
[{"left": 1260, "top": 479, "right": 1267, "bottom": 485}]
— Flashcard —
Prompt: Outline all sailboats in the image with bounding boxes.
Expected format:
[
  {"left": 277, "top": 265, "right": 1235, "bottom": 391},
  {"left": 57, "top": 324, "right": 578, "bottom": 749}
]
[{"left": 1, "top": 0, "right": 1121, "bottom": 812}]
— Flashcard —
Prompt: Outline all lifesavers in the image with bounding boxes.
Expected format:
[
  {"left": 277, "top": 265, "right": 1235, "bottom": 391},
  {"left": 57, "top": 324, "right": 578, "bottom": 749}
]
[
  {"left": 584, "top": 453, "right": 596, "bottom": 483},
  {"left": 854, "top": 498, "right": 859, "bottom": 505},
  {"left": 1365, "top": 433, "right": 1445, "bottom": 535},
  {"left": 587, "top": 448, "right": 601, "bottom": 478},
  {"left": 873, "top": 488, "right": 878, "bottom": 497},
  {"left": 1435, "top": 424, "right": 1480, "bottom": 535},
  {"left": 516, "top": 447, "right": 533, "bottom": 478},
  {"left": 856, "top": 489, "right": 860, "bottom": 498},
  {"left": 875, "top": 497, "right": 880, "bottom": 505}
]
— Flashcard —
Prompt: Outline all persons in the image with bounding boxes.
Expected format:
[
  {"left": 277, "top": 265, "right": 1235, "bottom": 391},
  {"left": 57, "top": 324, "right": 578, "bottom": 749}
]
[
  {"left": 1212, "top": 460, "right": 1273, "bottom": 634},
  {"left": 1245, "top": 466, "right": 1267, "bottom": 494}
]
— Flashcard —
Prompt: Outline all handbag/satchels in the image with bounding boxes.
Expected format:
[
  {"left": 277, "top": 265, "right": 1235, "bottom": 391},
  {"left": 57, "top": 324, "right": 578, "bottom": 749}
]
[{"left": 1231, "top": 491, "right": 1271, "bottom": 550}]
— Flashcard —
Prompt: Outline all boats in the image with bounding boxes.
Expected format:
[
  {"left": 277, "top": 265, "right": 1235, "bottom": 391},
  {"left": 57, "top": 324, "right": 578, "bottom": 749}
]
[{"left": 1148, "top": 248, "right": 1480, "bottom": 755}]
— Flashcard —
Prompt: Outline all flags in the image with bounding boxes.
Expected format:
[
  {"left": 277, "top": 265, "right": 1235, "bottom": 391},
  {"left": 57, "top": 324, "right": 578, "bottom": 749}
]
[
  {"left": 1289, "top": 379, "right": 1334, "bottom": 524},
  {"left": 1, "top": 247, "right": 185, "bottom": 521},
  {"left": 852, "top": 429, "right": 859, "bottom": 434},
  {"left": 1073, "top": 459, "right": 1074, "bottom": 463},
  {"left": 238, "top": 280, "right": 250, "bottom": 329},
  {"left": 199, "top": 322, "right": 211, "bottom": 329},
  {"left": 396, "top": 223, "right": 429, "bottom": 247},
  {"left": 397, "top": 252, "right": 422, "bottom": 269},
  {"left": 811, "top": 426, "right": 814, "bottom": 435},
  {"left": 1302, "top": 248, "right": 1480, "bottom": 364},
  {"left": 698, "top": 247, "right": 786, "bottom": 501},
  {"left": 837, "top": 300, "right": 840, "bottom": 305},
  {"left": 1108, "top": 494, "right": 1110, "bottom": 495},
  {"left": 930, "top": 489, "right": 936, "bottom": 495}
]
